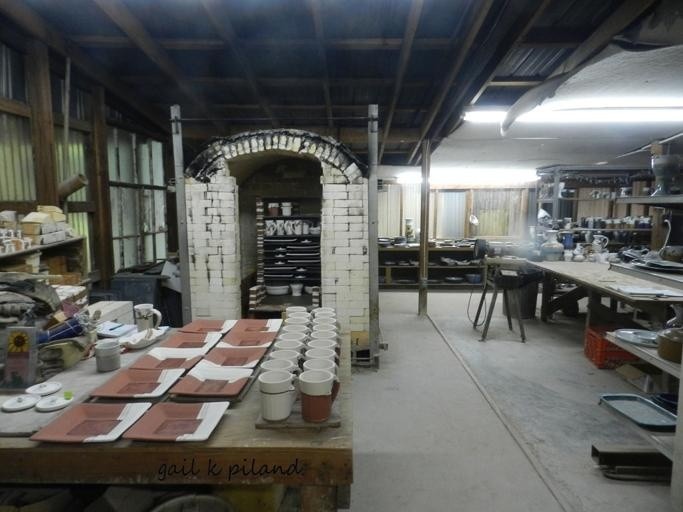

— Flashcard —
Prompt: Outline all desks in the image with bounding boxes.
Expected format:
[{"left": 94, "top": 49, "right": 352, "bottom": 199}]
[
  {"left": 484, "top": 254, "right": 527, "bottom": 289},
  {"left": 526, "top": 255, "right": 683, "bottom": 348},
  {"left": 0, "top": 327, "right": 353, "bottom": 510}
]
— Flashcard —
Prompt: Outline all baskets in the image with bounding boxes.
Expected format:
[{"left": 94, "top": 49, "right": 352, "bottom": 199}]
[{"left": 585, "top": 323, "right": 639, "bottom": 369}]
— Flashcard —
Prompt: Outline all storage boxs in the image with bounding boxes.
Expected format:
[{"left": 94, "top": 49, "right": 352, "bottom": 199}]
[{"left": 87, "top": 300, "right": 135, "bottom": 325}]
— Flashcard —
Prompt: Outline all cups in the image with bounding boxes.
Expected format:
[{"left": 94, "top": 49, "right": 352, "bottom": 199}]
[
  {"left": 649, "top": 153, "right": 682, "bottom": 197},
  {"left": 265, "top": 202, "right": 321, "bottom": 236},
  {"left": 658, "top": 245, "right": 683, "bottom": 261},
  {"left": 257, "top": 305, "right": 340, "bottom": 423},
  {"left": 530, "top": 215, "right": 651, "bottom": 262},
  {"left": 291, "top": 283, "right": 303, "bottom": 297},
  {"left": 133, "top": 303, "right": 162, "bottom": 332},
  {"left": 93, "top": 340, "right": 120, "bottom": 372}
]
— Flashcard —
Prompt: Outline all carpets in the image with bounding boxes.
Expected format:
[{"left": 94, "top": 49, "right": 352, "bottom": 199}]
[{"left": 425, "top": 311, "right": 671, "bottom": 511}]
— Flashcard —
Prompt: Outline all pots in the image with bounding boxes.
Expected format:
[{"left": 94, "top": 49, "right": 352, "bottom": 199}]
[{"left": 655, "top": 327, "right": 682, "bottom": 364}]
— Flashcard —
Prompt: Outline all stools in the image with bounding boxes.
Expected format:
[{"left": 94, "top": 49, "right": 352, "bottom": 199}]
[{"left": 473, "top": 269, "right": 526, "bottom": 343}]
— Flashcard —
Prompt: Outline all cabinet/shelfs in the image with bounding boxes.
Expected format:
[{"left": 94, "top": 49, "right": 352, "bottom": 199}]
[
  {"left": 597, "top": 194, "right": 683, "bottom": 503},
  {"left": 0, "top": 234, "right": 89, "bottom": 289},
  {"left": 378, "top": 245, "right": 485, "bottom": 288},
  {"left": 535, "top": 163, "right": 656, "bottom": 293},
  {"left": 261, "top": 195, "right": 321, "bottom": 286}
]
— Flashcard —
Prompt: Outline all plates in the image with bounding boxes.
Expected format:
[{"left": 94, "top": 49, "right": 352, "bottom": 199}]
[
  {"left": 2, "top": 394, "right": 40, "bottom": 411},
  {"left": 614, "top": 328, "right": 661, "bottom": 348},
  {"left": 35, "top": 394, "right": 72, "bottom": 411},
  {"left": 23, "top": 382, "right": 61, "bottom": 396},
  {"left": 166, "top": 367, "right": 253, "bottom": 397},
  {"left": 122, "top": 402, "right": 229, "bottom": 443},
  {"left": 129, "top": 318, "right": 282, "bottom": 369},
  {"left": 632, "top": 330, "right": 657, "bottom": 343},
  {"left": 116, "top": 325, "right": 169, "bottom": 349},
  {"left": 378, "top": 236, "right": 483, "bottom": 283},
  {"left": 650, "top": 392, "right": 679, "bottom": 412},
  {"left": 631, "top": 257, "right": 683, "bottom": 272},
  {"left": 89, "top": 368, "right": 185, "bottom": 400},
  {"left": 29, "top": 402, "right": 152, "bottom": 443}
]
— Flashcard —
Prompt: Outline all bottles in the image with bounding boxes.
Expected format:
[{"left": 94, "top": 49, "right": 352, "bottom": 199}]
[{"left": 404, "top": 218, "right": 415, "bottom": 242}]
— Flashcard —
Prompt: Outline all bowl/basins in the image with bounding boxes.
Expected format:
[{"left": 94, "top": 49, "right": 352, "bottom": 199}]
[
  {"left": 304, "top": 285, "right": 320, "bottom": 295},
  {"left": 263, "top": 285, "right": 290, "bottom": 296}
]
